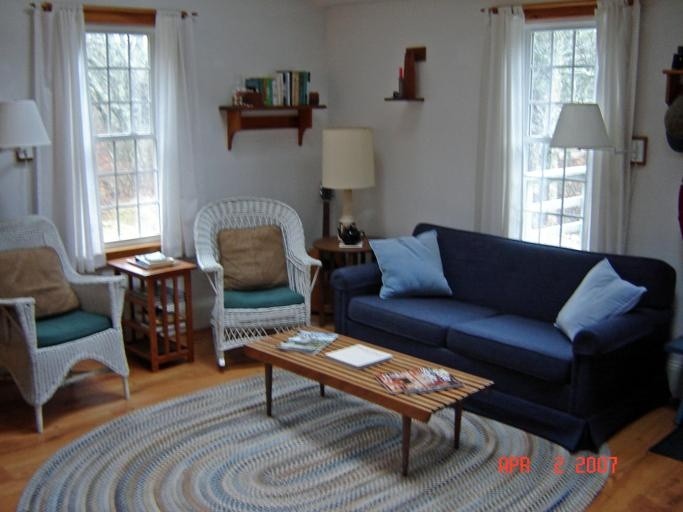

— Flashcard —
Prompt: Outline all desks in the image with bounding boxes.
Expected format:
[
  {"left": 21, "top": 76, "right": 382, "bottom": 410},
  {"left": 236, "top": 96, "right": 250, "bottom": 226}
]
[
  {"left": 105, "top": 253, "right": 198, "bottom": 372},
  {"left": 312, "top": 235, "right": 385, "bottom": 328}
]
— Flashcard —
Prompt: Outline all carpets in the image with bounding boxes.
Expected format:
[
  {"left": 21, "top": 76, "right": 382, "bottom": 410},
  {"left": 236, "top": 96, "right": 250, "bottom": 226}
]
[
  {"left": 648, "top": 425, "right": 682, "bottom": 463},
  {"left": 15, "top": 367, "right": 616, "bottom": 512}
]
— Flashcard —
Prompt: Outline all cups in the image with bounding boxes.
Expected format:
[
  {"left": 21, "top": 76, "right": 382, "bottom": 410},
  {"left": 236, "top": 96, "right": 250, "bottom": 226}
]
[{"left": 309, "top": 91, "right": 319, "bottom": 107}]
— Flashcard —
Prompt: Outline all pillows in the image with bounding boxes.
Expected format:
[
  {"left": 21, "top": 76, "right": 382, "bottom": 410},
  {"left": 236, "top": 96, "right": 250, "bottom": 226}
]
[
  {"left": 217, "top": 224, "right": 290, "bottom": 293},
  {"left": 367, "top": 229, "right": 455, "bottom": 300},
  {"left": 0, "top": 245, "right": 80, "bottom": 319},
  {"left": 553, "top": 257, "right": 648, "bottom": 345}
]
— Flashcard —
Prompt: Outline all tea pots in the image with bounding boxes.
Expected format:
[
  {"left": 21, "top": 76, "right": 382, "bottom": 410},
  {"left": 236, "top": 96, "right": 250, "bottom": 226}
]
[{"left": 336, "top": 223, "right": 366, "bottom": 245}]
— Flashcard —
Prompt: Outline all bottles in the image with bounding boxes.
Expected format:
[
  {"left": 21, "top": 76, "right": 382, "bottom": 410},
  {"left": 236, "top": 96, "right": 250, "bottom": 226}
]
[{"left": 232, "top": 73, "right": 245, "bottom": 107}]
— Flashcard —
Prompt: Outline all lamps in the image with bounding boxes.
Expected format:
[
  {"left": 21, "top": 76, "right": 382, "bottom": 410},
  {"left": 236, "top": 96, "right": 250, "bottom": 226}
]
[
  {"left": 549, "top": 101, "right": 648, "bottom": 166},
  {"left": 0, "top": 99, "right": 52, "bottom": 161},
  {"left": 321, "top": 126, "right": 380, "bottom": 242}
]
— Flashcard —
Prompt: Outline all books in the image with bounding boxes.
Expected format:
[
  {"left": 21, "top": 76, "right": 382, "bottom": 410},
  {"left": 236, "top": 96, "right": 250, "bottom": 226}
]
[
  {"left": 276, "top": 328, "right": 338, "bottom": 356},
  {"left": 374, "top": 367, "right": 463, "bottom": 395},
  {"left": 245, "top": 69, "right": 312, "bottom": 106},
  {"left": 127, "top": 250, "right": 174, "bottom": 269},
  {"left": 325, "top": 343, "right": 393, "bottom": 369}
]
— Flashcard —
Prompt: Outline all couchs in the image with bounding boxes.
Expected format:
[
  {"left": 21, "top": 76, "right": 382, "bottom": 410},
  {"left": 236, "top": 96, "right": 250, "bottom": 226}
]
[{"left": 329, "top": 221, "right": 678, "bottom": 455}]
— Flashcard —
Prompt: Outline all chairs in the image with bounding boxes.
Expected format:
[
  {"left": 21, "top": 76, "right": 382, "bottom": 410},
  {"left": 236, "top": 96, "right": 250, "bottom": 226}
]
[
  {"left": 0, "top": 214, "right": 133, "bottom": 434},
  {"left": 192, "top": 194, "right": 323, "bottom": 368}
]
